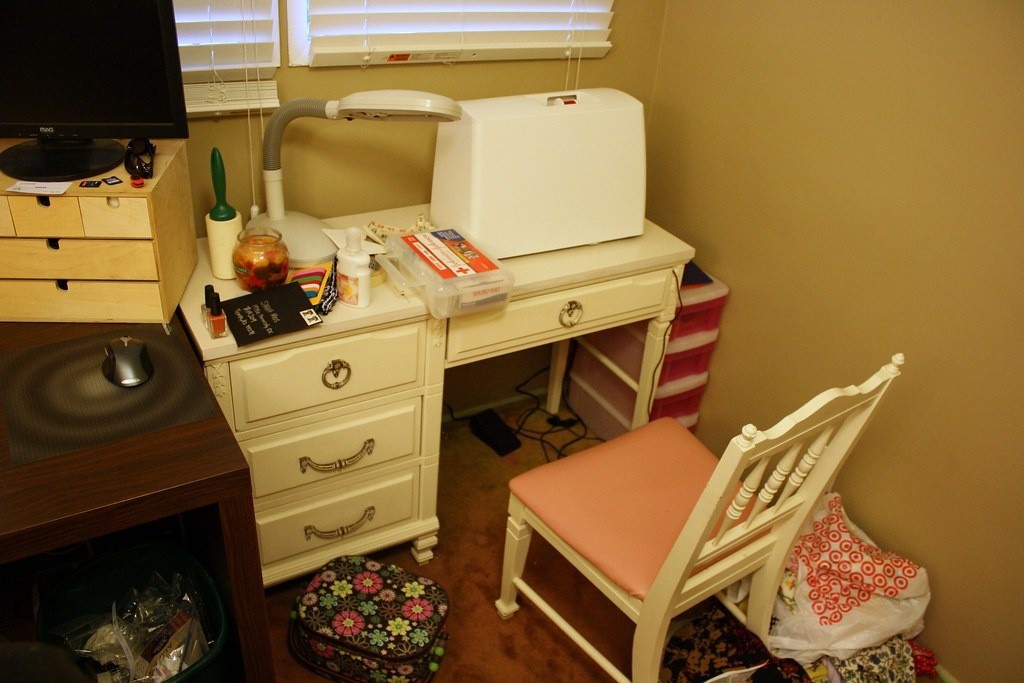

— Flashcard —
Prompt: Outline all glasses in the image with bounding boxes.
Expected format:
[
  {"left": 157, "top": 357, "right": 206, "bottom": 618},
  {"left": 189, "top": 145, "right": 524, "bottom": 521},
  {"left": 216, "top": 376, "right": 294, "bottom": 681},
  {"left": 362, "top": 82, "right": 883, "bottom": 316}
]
[{"left": 124, "top": 135, "right": 157, "bottom": 179}]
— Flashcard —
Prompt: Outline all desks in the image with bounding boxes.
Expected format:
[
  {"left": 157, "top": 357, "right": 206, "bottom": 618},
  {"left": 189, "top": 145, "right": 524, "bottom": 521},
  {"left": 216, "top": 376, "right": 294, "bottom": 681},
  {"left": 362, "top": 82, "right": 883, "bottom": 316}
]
[
  {"left": 181, "top": 199, "right": 695, "bottom": 587},
  {"left": 0, "top": 322, "right": 277, "bottom": 683}
]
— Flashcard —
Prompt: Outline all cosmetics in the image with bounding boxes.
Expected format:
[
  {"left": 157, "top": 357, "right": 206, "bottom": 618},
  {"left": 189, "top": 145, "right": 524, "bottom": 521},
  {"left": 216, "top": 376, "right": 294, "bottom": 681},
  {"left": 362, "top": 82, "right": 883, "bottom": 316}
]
[{"left": 336, "top": 228, "right": 370, "bottom": 308}]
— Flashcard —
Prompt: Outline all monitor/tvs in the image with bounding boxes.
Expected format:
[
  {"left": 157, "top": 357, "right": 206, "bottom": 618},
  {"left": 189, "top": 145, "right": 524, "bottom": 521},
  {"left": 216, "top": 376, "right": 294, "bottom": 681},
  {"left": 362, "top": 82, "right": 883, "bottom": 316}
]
[{"left": 0, "top": 0, "right": 190, "bottom": 182}]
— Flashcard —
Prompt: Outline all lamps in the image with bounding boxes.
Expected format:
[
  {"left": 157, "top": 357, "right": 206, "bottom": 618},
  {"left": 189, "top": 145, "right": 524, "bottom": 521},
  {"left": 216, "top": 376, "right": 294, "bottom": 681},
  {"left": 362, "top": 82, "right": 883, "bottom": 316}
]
[{"left": 244, "top": 90, "right": 464, "bottom": 267}]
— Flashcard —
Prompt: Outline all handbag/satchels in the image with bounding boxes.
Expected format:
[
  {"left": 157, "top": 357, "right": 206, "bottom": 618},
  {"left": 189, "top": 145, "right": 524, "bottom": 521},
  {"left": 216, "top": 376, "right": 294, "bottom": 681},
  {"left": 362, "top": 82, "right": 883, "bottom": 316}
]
[
  {"left": 725, "top": 492, "right": 931, "bottom": 665},
  {"left": 286, "top": 555, "right": 450, "bottom": 683}
]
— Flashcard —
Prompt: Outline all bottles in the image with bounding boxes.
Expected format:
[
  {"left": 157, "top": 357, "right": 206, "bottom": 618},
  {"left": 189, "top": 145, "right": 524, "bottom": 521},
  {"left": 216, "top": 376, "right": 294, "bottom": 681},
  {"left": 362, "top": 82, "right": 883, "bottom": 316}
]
[{"left": 232, "top": 227, "right": 290, "bottom": 292}]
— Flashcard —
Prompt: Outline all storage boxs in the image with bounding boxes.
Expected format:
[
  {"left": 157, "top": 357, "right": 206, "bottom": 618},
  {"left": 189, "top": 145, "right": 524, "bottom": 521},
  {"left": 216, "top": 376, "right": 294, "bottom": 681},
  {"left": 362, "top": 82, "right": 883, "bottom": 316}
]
[
  {"left": 678, "top": 412, "right": 701, "bottom": 434},
  {"left": 657, "top": 328, "right": 721, "bottom": 389},
  {"left": 284, "top": 556, "right": 452, "bottom": 683},
  {"left": 667, "top": 269, "right": 731, "bottom": 342},
  {"left": 393, "top": 226, "right": 516, "bottom": 321},
  {"left": 649, "top": 371, "right": 710, "bottom": 423}
]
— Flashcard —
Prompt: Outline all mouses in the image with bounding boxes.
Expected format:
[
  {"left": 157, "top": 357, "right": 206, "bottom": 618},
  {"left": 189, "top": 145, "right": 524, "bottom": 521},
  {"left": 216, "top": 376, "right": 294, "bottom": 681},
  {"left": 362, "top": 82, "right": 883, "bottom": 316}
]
[{"left": 102, "top": 335, "right": 154, "bottom": 388}]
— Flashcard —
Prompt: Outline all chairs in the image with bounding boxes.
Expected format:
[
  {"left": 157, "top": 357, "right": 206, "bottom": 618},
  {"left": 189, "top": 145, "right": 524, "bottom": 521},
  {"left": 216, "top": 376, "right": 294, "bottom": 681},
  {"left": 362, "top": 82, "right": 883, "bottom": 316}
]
[{"left": 501, "top": 352, "right": 907, "bottom": 683}]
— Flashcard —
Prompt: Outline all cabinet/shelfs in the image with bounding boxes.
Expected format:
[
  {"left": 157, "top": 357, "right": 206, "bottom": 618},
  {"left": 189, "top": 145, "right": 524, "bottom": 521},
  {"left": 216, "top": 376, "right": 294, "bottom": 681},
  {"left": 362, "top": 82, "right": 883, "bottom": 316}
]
[{"left": 0, "top": 139, "right": 200, "bottom": 338}]
